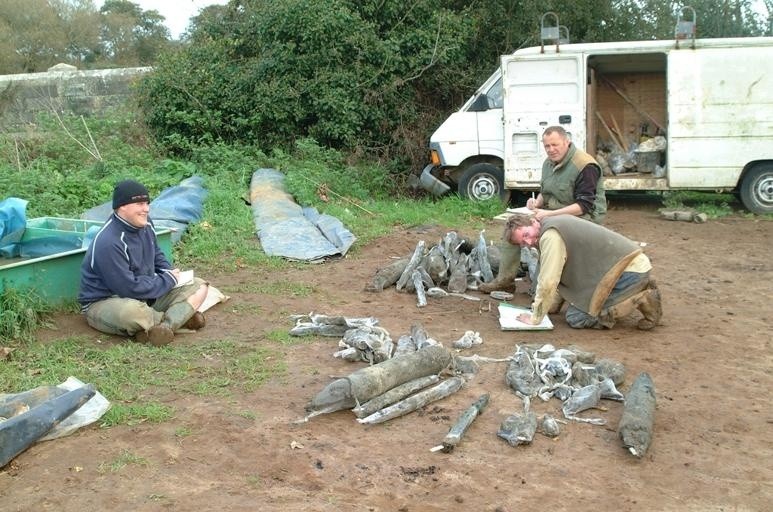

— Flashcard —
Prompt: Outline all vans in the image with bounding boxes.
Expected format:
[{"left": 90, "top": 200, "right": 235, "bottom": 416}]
[{"left": 420, "top": 6, "right": 773, "bottom": 216}]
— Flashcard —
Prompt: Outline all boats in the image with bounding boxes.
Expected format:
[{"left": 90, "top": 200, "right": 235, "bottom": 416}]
[{"left": 0, "top": 217, "right": 180, "bottom": 305}]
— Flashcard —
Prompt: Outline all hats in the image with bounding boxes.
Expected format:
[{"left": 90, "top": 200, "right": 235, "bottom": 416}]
[{"left": 112, "top": 180, "right": 151, "bottom": 209}]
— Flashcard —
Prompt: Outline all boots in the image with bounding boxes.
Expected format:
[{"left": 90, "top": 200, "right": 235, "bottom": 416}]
[
  {"left": 134, "top": 300, "right": 207, "bottom": 346},
  {"left": 478, "top": 238, "right": 541, "bottom": 294},
  {"left": 597, "top": 279, "right": 663, "bottom": 331}
]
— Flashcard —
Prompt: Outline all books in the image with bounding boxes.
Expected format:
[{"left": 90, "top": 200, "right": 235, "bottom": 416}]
[
  {"left": 497, "top": 302, "right": 553, "bottom": 331},
  {"left": 174, "top": 269, "right": 194, "bottom": 287},
  {"left": 506, "top": 205, "right": 535, "bottom": 215}
]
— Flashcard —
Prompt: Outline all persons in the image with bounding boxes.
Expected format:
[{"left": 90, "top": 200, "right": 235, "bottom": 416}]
[
  {"left": 477, "top": 126, "right": 607, "bottom": 294},
  {"left": 503, "top": 214, "right": 663, "bottom": 331},
  {"left": 76, "top": 179, "right": 208, "bottom": 346}
]
